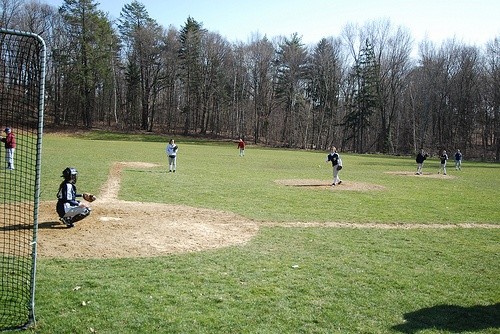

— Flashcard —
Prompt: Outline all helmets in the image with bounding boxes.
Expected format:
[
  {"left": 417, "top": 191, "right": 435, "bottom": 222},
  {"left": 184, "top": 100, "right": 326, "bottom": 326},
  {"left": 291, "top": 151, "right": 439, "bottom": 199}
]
[{"left": 60, "top": 168, "right": 78, "bottom": 177}]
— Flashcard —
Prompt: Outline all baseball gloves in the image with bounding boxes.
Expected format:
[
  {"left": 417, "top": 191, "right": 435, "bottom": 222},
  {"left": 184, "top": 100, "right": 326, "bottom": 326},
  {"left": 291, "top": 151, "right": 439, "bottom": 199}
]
[
  {"left": 83, "top": 193, "right": 97, "bottom": 203},
  {"left": 337, "top": 166, "right": 342, "bottom": 171}
]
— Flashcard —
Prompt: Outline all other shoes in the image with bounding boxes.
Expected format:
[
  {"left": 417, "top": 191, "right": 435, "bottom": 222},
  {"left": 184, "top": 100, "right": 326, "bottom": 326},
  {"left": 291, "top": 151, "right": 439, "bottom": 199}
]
[
  {"left": 67, "top": 223, "right": 75, "bottom": 227},
  {"left": 59, "top": 217, "right": 67, "bottom": 224}
]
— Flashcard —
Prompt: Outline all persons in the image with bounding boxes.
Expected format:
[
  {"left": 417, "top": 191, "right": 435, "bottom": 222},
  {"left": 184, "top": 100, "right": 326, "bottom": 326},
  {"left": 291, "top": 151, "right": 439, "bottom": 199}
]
[
  {"left": 237, "top": 139, "right": 245, "bottom": 157},
  {"left": 453, "top": 149, "right": 462, "bottom": 171},
  {"left": 55, "top": 166, "right": 98, "bottom": 227},
  {"left": 325, "top": 146, "right": 343, "bottom": 186},
  {"left": 166, "top": 139, "right": 179, "bottom": 173},
  {"left": 438, "top": 150, "right": 449, "bottom": 175},
  {"left": 0, "top": 128, "right": 17, "bottom": 170},
  {"left": 416, "top": 149, "right": 430, "bottom": 175}
]
